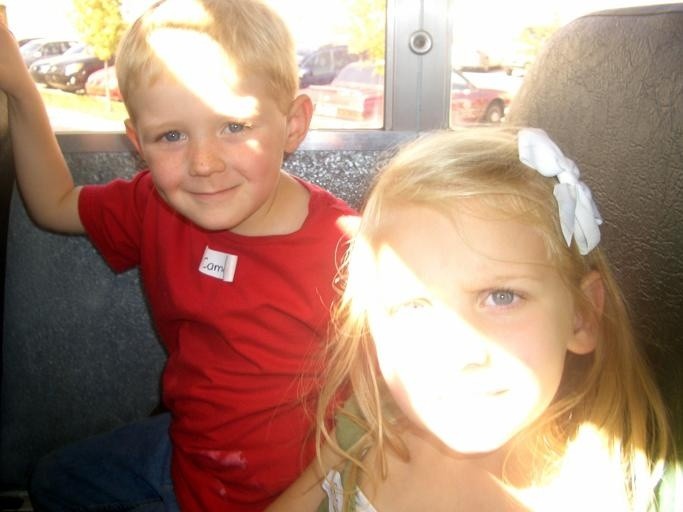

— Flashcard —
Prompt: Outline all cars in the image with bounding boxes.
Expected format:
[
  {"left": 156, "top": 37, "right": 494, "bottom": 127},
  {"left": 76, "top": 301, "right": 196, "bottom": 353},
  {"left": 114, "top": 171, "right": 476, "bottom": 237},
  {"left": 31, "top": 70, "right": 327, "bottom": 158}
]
[
  {"left": 297, "top": 42, "right": 371, "bottom": 89},
  {"left": 305, "top": 55, "right": 510, "bottom": 127},
  {"left": 13, "top": 36, "right": 124, "bottom": 101}
]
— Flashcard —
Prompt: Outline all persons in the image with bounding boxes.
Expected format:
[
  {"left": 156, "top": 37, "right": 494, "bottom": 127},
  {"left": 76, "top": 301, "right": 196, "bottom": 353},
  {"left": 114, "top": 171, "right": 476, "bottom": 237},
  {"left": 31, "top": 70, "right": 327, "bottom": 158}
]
[
  {"left": 263, "top": 125, "right": 682, "bottom": 512},
  {"left": 1, "top": 0, "right": 381, "bottom": 512}
]
